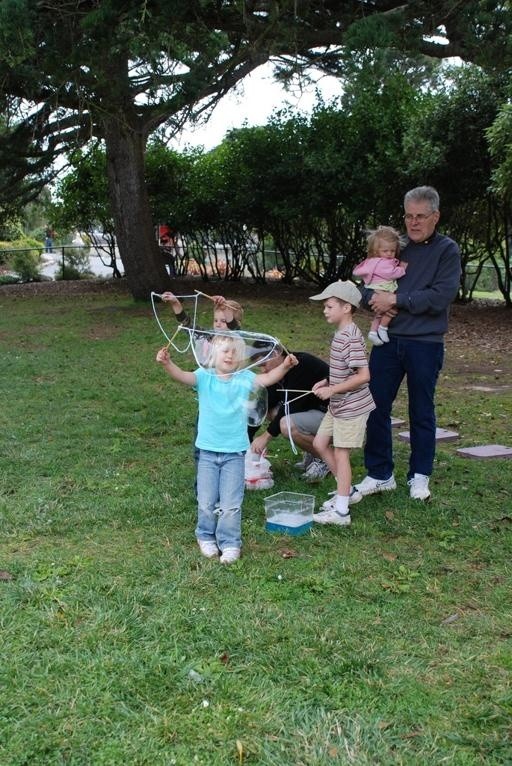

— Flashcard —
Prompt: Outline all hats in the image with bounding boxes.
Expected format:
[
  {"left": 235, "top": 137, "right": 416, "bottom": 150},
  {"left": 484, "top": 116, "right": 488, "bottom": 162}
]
[{"left": 308, "top": 280, "right": 363, "bottom": 309}]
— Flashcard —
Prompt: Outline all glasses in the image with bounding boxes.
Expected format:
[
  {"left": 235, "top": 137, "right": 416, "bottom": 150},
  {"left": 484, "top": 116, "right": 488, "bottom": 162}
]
[{"left": 402, "top": 211, "right": 435, "bottom": 222}]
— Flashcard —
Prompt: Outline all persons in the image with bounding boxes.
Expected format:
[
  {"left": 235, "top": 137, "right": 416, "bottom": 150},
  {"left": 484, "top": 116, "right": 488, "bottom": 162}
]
[
  {"left": 309, "top": 280, "right": 377, "bottom": 525},
  {"left": 161, "top": 290, "right": 245, "bottom": 507},
  {"left": 155, "top": 329, "right": 300, "bottom": 562},
  {"left": 246, "top": 336, "right": 331, "bottom": 482},
  {"left": 355, "top": 184, "right": 462, "bottom": 499},
  {"left": 352, "top": 225, "right": 408, "bottom": 346},
  {"left": 45, "top": 222, "right": 56, "bottom": 252}
]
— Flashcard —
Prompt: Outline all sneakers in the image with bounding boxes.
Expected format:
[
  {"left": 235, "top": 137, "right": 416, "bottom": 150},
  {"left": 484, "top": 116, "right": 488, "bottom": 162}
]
[
  {"left": 293, "top": 451, "right": 315, "bottom": 470},
  {"left": 311, "top": 508, "right": 351, "bottom": 527},
  {"left": 298, "top": 456, "right": 330, "bottom": 484},
  {"left": 219, "top": 547, "right": 240, "bottom": 566},
  {"left": 405, "top": 473, "right": 432, "bottom": 502},
  {"left": 321, "top": 485, "right": 363, "bottom": 510},
  {"left": 194, "top": 538, "right": 220, "bottom": 559},
  {"left": 353, "top": 472, "right": 398, "bottom": 497}
]
